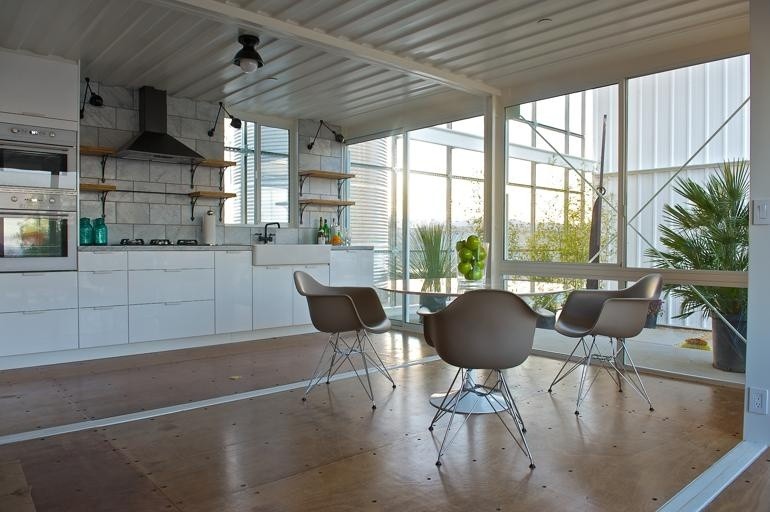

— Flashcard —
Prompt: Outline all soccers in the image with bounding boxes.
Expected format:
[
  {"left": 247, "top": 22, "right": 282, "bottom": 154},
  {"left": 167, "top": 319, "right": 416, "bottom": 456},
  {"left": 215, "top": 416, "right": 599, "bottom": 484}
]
[{"left": 456, "top": 235, "right": 487, "bottom": 280}]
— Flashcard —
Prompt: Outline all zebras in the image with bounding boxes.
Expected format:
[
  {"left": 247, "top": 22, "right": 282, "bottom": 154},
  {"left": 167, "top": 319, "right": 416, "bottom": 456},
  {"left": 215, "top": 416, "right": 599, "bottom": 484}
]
[{"left": 0, "top": 140, "right": 76, "bottom": 272}]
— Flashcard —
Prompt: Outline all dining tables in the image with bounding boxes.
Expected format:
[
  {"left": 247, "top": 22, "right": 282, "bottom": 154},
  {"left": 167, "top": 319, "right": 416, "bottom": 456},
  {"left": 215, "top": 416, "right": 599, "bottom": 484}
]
[
  {"left": 645, "top": 300, "right": 663, "bottom": 328},
  {"left": 531, "top": 295, "right": 556, "bottom": 331},
  {"left": 384, "top": 222, "right": 464, "bottom": 323},
  {"left": 643, "top": 157, "right": 750, "bottom": 373}
]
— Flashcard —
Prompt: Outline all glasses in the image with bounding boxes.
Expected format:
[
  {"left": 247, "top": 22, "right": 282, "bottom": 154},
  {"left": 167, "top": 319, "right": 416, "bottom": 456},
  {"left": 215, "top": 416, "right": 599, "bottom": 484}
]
[
  {"left": 80, "top": 77, "right": 103, "bottom": 119},
  {"left": 234, "top": 35, "right": 264, "bottom": 73},
  {"left": 307, "top": 120, "right": 345, "bottom": 150},
  {"left": 207, "top": 102, "right": 241, "bottom": 137}
]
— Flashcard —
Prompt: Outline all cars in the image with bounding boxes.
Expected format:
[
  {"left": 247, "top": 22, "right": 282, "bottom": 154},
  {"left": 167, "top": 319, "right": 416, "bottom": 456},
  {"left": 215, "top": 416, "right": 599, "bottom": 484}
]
[{"left": 341, "top": 233, "right": 353, "bottom": 247}]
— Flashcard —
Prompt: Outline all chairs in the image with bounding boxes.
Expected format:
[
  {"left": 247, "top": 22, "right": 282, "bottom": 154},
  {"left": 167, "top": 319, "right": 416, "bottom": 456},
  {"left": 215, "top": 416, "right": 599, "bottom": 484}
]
[
  {"left": 549, "top": 273, "right": 663, "bottom": 416},
  {"left": 418, "top": 289, "right": 555, "bottom": 469},
  {"left": 293, "top": 270, "right": 396, "bottom": 410}
]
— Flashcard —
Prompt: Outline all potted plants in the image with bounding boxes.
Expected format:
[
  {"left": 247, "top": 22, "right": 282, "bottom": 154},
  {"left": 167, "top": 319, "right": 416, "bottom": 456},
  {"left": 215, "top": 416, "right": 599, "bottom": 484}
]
[
  {"left": 531, "top": 295, "right": 556, "bottom": 331},
  {"left": 645, "top": 300, "right": 663, "bottom": 328},
  {"left": 384, "top": 222, "right": 464, "bottom": 323},
  {"left": 643, "top": 157, "right": 750, "bottom": 373}
]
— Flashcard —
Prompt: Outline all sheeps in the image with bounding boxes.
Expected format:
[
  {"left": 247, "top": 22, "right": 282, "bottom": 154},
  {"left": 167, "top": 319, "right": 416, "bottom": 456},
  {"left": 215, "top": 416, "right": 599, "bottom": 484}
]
[{"left": 265, "top": 223, "right": 280, "bottom": 243}]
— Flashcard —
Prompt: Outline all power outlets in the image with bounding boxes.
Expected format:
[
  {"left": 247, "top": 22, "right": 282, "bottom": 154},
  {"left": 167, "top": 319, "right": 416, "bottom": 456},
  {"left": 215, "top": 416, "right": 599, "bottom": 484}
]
[{"left": 749, "top": 386, "right": 768, "bottom": 414}]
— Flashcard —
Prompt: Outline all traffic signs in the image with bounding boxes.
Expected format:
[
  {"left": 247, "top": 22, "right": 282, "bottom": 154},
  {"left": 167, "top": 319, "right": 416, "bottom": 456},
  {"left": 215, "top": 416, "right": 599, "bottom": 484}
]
[{"left": 749, "top": 386, "right": 768, "bottom": 414}]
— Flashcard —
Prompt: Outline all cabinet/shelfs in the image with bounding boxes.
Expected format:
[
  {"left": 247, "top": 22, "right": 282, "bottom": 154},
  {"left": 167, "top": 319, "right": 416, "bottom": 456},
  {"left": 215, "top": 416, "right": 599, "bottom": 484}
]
[
  {"left": 317, "top": 217, "right": 326, "bottom": 244},
  {"left": 80, "top": 218, "right": 92, "bottom": 246},
  {"left": 189, "top": 161, "right": 236, "bottom": 221},
  {"left": 0, "top": 251, "right": 374, "bottom": 371},
  {"left": 298, "top": 171, "right": 355, "bottom": 224},
  {"left": 202, "top": 211, "right": 217, "bottom": 245},
  {"left": 80, "top": 146, "right": 116, "bottom": 218},
  {"left": 93, "top": 217, "right": 108, "bottom": 246},
  {"left": 0, "top": 48, "right": 81, "bottom": 122},
  {"left": 323, "top": 218, "right": 340, "bottom": 244}
]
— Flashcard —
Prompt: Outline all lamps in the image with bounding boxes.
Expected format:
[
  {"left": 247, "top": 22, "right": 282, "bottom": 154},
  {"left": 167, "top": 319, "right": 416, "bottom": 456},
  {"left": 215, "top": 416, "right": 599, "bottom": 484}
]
[
  {"left": 307, "top": 120, "right": 345, "bottom": 150},
  {"left": 80, "top": 77, "right": 103, "bottom": 119},
  {"left": 207, "top": 102, "right": 241, "bottom": 137},
  {"left": 418, "top": 289, "right": 555, "bottom": 469},
  {"left": 293, "top": 270, "right": 396, "bottom": 410},
  {"left": 549, "top": 273, "right": 663, "bottom": 416},
  {"left": 234, "top": 35, "right": 264, "bottom": 73}
]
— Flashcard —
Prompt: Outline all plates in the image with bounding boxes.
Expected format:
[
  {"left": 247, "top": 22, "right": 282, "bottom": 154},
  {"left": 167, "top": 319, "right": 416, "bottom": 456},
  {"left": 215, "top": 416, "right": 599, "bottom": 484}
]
[
  {"left": 189, "top": 161, "right": 236, "bottom": 221},
  {"left": 298, "top": 171, "right": 355, "bottom": 224},
  {"left": 80, "top": 146, "right": 116, "bottom": 218},
  {"left": 0, "top": 48, "right": 81, "bottom": 122}
]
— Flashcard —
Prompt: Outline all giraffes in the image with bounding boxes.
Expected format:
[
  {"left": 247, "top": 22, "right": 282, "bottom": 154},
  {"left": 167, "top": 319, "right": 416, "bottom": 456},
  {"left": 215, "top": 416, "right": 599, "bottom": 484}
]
[{"left": 111, "top": 239, "right": 200, "bottom": 248}]
